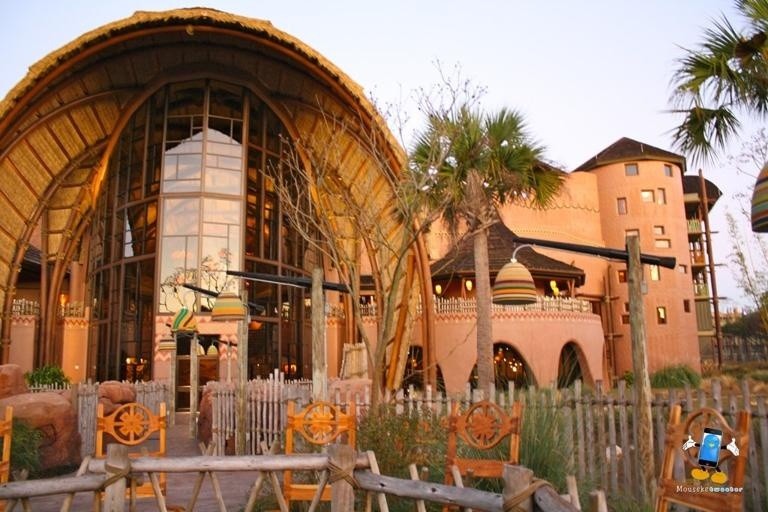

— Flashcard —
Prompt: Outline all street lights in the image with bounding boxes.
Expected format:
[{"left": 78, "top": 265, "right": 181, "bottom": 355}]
[
  {"left": 492, "top": 227, "right": 677, "bottom": 509},
  {"left": 209, "top": 262, "right": 351, "bottom": 483},
  {"left": 171, "top": 284, "right": 266, "bottom": 457}
]
